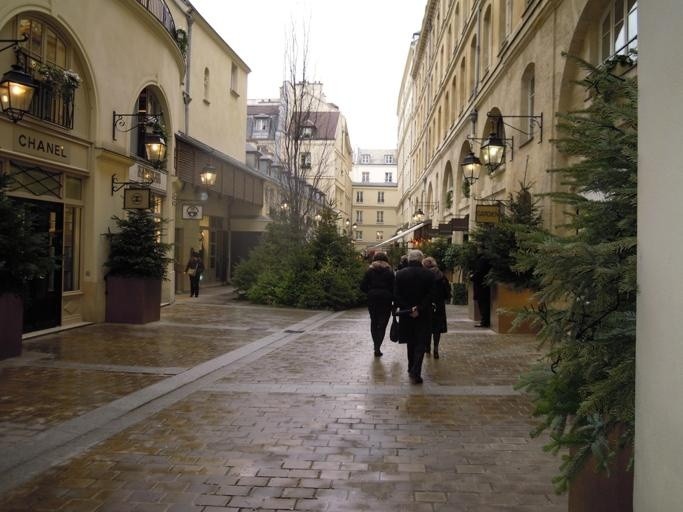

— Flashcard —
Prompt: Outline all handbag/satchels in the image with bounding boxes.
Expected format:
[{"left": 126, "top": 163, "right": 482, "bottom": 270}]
[
  {"left": 390, "top": 322, "right": 399, "bottom": 342},
  {"left": 187, "top": 268, "right": 196, "bottom": 277}
]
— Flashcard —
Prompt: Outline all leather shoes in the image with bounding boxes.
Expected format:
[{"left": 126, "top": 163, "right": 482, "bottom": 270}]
[{"left": 425, "top": 345, "right": 439, "bottom": 359}]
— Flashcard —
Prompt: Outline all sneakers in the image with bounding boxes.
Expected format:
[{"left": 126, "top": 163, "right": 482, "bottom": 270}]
[{"left": 411, "top": 375, "right": 423, "bottom": 385}]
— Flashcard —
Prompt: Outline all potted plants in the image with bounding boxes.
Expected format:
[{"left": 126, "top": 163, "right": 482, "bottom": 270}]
[
  {"left": 464, "top": 178, "right": 555, "bottom": 334},
  {"left": 508, "top": 48, "right": 638, "bottom": 512},
  {"left": 101, "top": 201, "right": 182, "bottom": 325},
  {"left": 0, "top": 171, "right": 57, "bottom": 360},
  {"left": 443, "top": 244, "right": 472, "bottom": 307}
]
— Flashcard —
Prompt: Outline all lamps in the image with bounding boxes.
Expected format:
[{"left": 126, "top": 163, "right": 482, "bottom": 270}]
[
  {"left": 480, "top": 111, "right": 544, "bottom": 176},
  {"left": 195, "top": 147, "right": 220, "bottom": 191},
  {"left": 112, "top": 111, "right": 168, "bottom": 171},
  {"left": 0, "top": 39, "right": 37, "bottom": 125},
  {"left": 460, "top": 133, "right": 516, "bottom": 185},
  {"left": 414, "top": 207, "right": 425, "bottom": 219}
]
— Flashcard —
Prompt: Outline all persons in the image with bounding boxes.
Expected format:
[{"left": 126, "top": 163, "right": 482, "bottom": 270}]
[
  {"left": 183, "top": 252, "right": 205, "bottom": 297},
  {"left": 360, "top": 249, "right": 454, "bottom": 384}
]
